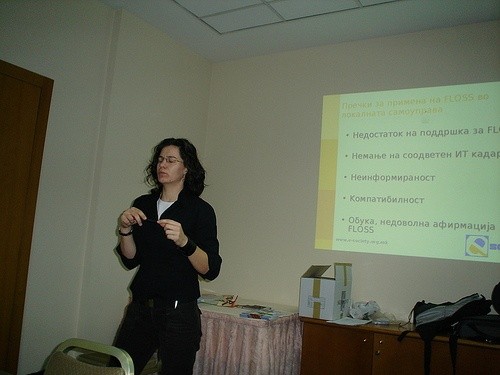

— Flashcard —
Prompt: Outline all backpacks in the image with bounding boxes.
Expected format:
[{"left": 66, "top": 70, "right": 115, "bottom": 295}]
[{"left": 397, "top": 292, "right": 493, "bottom": 375}]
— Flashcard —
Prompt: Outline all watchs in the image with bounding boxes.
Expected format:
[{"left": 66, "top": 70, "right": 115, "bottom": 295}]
[{"left": 118, "top": 226, "right": 135, "bottom": 236}]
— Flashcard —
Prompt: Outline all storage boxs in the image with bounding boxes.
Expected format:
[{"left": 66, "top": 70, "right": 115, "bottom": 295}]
[{"left": 298, "top": 263, "right": 352, "bottom": 320}]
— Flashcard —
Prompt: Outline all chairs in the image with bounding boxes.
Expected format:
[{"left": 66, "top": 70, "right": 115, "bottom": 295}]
[{"left": 43, "top": 338, "right": 135, "bottom": 375}]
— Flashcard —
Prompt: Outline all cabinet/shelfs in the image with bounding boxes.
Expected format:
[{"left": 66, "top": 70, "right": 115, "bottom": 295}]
[{"left": 298, "top": 317, "right": 500, "bottom": 375}]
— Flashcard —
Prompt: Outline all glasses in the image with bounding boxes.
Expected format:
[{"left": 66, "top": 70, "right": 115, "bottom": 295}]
[{"left": 157, "top": 156, "right": 184, "bottom": 164}]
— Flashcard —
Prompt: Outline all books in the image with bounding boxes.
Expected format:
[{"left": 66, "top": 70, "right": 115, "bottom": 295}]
[
  {"left": 201, "top": 295, "right": 282, "bottom": 321},
  {"left": 371, "top": 317, "right": 402, "bottom": 325}
]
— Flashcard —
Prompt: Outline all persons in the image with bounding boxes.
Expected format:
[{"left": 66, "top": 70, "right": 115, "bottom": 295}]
[{"left": 105, "top": 137, "right": 223, "bottom": 375}]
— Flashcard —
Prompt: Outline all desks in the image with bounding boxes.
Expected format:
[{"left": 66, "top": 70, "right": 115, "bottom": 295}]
[{"left": 193, "top": 297, "right": 304, "bottom": 375}]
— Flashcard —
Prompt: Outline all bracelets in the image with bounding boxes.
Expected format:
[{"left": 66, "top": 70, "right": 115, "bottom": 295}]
[{"left": 178, "top": 235, "right": 197, "bottom": 257}]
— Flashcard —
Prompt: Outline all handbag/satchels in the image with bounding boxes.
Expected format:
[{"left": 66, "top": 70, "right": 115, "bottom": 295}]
[{"left": 451, "top": 314, "right": 500, "bottom": 345}]
